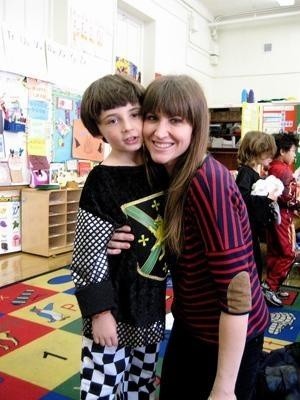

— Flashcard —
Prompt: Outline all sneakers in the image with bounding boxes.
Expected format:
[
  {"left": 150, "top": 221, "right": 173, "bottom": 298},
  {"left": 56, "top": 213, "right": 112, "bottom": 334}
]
[
  {"left": 259, "top": 282, "right": 282, "bottom": 309},
  {"left": 275, "top": 288, "right": 290, "bottom": 299}
]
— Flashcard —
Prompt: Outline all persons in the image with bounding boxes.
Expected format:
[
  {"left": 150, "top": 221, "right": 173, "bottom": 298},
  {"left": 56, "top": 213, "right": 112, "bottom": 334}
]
[
  {"left": 69, "top": 75, "right": 172, "bottom": 399},
  {"left": 236, "top": 131, "right": 278, "bottom": 283},
  {"left": 106, "top": 74, "right": 271, "bottom": 400},
  {"left": 261, "top": 133, "right": 300, "bottom": 307}
]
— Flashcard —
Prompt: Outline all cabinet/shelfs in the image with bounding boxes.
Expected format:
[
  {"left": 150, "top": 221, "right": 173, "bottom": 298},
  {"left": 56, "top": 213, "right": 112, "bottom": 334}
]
[{"left": 20, "top": 188, "right": 82, "bottom": 257}]
